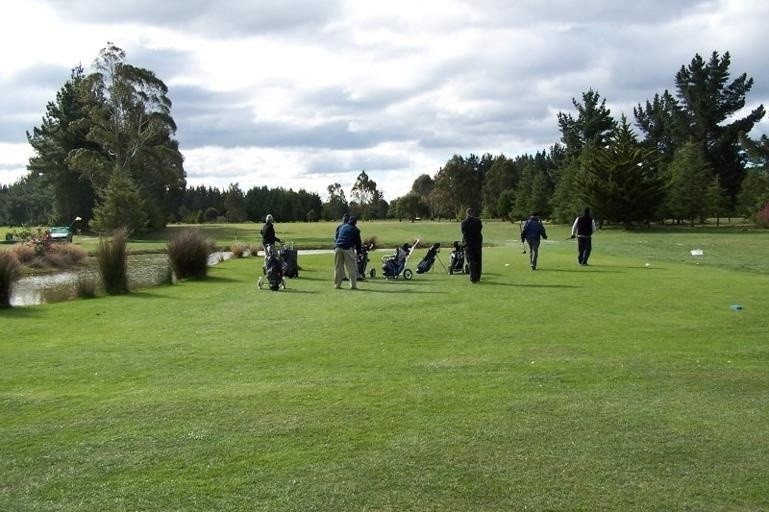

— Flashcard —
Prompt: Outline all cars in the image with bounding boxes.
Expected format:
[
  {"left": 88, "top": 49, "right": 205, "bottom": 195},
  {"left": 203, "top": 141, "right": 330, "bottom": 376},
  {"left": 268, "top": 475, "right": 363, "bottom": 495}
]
[{"left": 49, "top": 225, "right": 74, "bottom": 243}]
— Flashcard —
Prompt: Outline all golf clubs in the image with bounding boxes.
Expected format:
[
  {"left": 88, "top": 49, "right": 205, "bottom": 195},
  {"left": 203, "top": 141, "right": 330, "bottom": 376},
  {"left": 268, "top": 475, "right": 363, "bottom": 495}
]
[
  {"left": 519, "top": 224, "right": 526, "bottom": 254},
  {"left": 292, "top": 241, "right": 295, "bottom": 250},
  {"left": 566, "top": 236, "right": 577, "bottom": 240}
]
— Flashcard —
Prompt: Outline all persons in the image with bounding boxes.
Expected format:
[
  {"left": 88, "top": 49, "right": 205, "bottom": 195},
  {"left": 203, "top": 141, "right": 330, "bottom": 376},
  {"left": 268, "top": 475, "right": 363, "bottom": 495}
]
[
  {"left": 334, "top": 213, "right": 365, "bottom": 281},
  {"left": 26, "top": 227, "right": 53, "bottom": 248},
  {"left": 570, "top": 206, "right": 597, "bottom": 266},
  {"left": 333, "top": 215, "right": 362, "bottom": 289},
  {"left": 259, "top": 213, "right": 283, "bottom": 276},
  {"left": 460, "top": 206, "right": 484, "bottom": 282},
  {"left": 520, "top": 211, "right": 548, "bottom": 270}
]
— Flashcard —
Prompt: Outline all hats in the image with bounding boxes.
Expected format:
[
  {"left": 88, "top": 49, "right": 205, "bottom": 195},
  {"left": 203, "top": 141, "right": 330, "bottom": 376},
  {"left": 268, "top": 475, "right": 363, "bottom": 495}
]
[{"left": 343, "top": 213, "right": 358, "bottom": 223}]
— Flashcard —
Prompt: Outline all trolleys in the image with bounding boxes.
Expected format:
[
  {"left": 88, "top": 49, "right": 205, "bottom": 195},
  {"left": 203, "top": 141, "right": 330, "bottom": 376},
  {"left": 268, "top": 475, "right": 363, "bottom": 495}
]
[
  {"left": 381, "top": 238, "right": 420, "bottom": 281},
  {"left": 257, "top": 243, "right": 287, "bottom": 291},
  {"left": 354, "top": 241, "right": 376, "bottom": 280},
  {"left": 448, "top": 241, "right": 470, "bottom": 275},
  {"left": 278, "top": 242, "right": 299, "bottom": 278}
]
